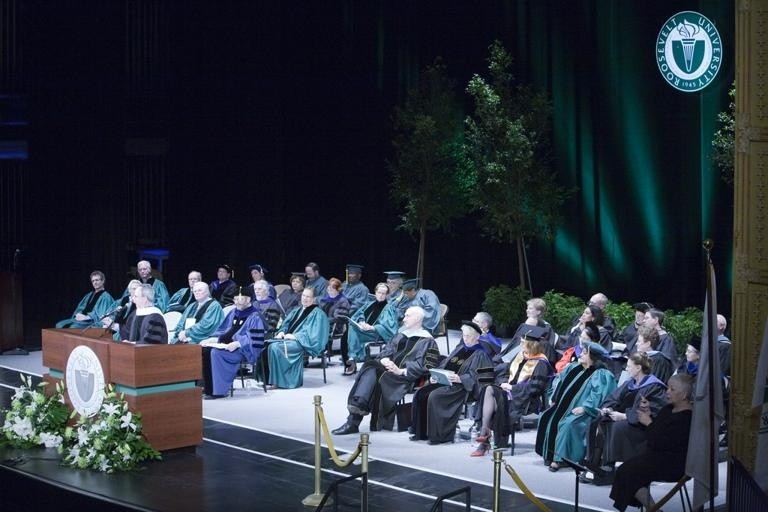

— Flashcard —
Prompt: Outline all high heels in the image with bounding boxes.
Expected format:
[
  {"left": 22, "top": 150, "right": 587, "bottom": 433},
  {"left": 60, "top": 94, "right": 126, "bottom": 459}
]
[
  {"left": 470, "top": 443, "right": 491, "bottom": 457},
  {"left": 474, "top": 429, "right": 493, "bottom": 442}
]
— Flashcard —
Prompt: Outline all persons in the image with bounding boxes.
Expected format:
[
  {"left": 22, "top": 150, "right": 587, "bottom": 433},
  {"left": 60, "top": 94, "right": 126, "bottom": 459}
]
[
  {"left": 341, "top": 264, "right": 370, "bottom": 317},
  {"left": 621, "top": 302, "right": 654, "bottom": 355},
  {"left": 492, "top": 298, "right": 554, "bottom": 365},
  {"left": 715, "top": 313, "right": 731, "bottom": 386},
  {"left": 170, "top": 282, "right": 226, "bottom": 344},
  {"left": 120, "top": 284, "right": 168, "bottom": 344},
  {"left": 571, "top": 292, "right": 616, "bottom": 339},
  {"left": 209, "top": 264, "right": 239, "bottom": 309},
  {"left": 470, "top": 325, "right": 554, "bottom": 457},
  {"left": 305, "top": 262, "right": 328, "bottom": 298},
  {"left": 396, "top": 278, "right": 440, "bottom": 339},
  {"left": 331, "top": 306, "right": 440, "bottom": 436},
  {"left": 277, "top": 275, "right": 306, "bottom": 317},
  {"left": 383, "top": 271, "right": 408, "bottom": 308},
  {"left": 560, "top": 305, "right": 613, "bottom": 354},
  {"left": 636, "top": 324, "right": 677, "bottom": 386},
  {"left": 164, "top": 271, "right": 202, "bottom": 313},
  {"left": 342, "top": 283, "right": 398, "bottom": 376},
  {"left": 578, "top": 352, "right": 669, "bottom": 486},
  {"left": 248, "top": 264, "right": 277, "bottom": 300},
  {"left": 55, "top": 271, "right": 114, "bottom": 329},
  {"left": 318, "top": 277, "right": 350, "bottom": 357},
  {"left": 672, "top": 338, "right": 728, "bottom": 389},
  {"left": 642, "top": 308, "right": 677, "bottom": 362},
  {"left": 122, "top": 260, "right": 169, "bottom": 315},
  {"left": 99, "top": 279, "right": 142, "bottom": 334},
  {"left": 252, "top": 280, "right": 280, "bottom": 337},
  {"left": 535, "top": 338, "right": 616, "bottom": 473},
  {"left": 200, "top": 286, "right": 266, "bottom": 400},
  {"left": 409, "top": 320, "right": 494, "bottom": 445},
  {"left": 609, "top": 374, "right": 693, "bottom": 512},
  {"left": 256, "top": 286, "right": 329, "bottom": 389},
  {"left": 456, "top": 312, "right": 502, "bottom": 362},
  {"left": 555, "top": 322, "right": 601, "bottom": 375}
]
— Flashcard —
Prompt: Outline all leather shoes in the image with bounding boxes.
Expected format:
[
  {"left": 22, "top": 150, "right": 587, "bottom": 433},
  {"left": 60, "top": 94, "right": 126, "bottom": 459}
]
[
  {"left": 550, "top": 462, "right": 560, "bottom": 473},
  {"left": 458, "top": 432, "right": 471, "bottom": 439},
  {"left": 342, "top": 367, "right": 357, "bottom": 376},
  {"left": 237, "top": 368, "right": 250, "bottom": 376},
  {"left": 578, "top": 469, "right": 596, "bottom": 485},
  {"left": 719, "top": 438, "right": 731, "bottom": 445},
  {"left": 427, "top": 439, "right": 448, "bottom": 444},
  {"left": 469, "top": 421, "right": 483, "bottom": 433},
  {"left": 346, "top": 401, "right": 371, "bottom": 418},
  {"left": 205, "top": 395, "right": 226, "bottom": 401},
  {"left": 331, "top": 423, "right": 359, "bottom": 435},
  {"left": 347, "top": 358, "right": 353, "bottom": 364},
  {"left": 718, "top": 423, "right": 728, "bottom": 432},
  {"left": 407, "top": 424, "right": 413, "bottom": 431}
]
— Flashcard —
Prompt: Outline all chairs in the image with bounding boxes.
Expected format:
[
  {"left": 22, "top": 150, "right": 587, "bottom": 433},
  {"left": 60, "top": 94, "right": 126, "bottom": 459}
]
[
  {"left": 227, "top": 350, "right": 268, "bottom": 397},
  {"left": 507, "top": 376, "right": 553, "bottom": 453},
  {"left": 436, "top": 317, "right": 450, "bottom": 354},
  {"left": 298, "top": 347, "right": 327, "bottom": 386},
  {"left": 640, "top": 477, "right": 693, "bottom": 512}
]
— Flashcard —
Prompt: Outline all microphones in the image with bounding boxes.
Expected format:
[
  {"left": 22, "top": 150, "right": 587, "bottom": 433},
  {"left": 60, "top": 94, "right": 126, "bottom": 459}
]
[
  {"left": 103, "top": 305, "right": 122, "bottom": 318},
  {"left": 116, "top": 303, "right": 130, "bottom": 317}
]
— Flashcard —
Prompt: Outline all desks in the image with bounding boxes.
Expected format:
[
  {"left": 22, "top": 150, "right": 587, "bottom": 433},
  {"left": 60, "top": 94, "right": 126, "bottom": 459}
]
[{"left": 41, "top": 328, "right": 203, "bottom": 452}]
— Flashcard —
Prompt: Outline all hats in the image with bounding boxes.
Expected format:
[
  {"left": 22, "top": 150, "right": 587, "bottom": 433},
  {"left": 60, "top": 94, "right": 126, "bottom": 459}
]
[
  {"left": 292, "top": 270, "right": 309, "bottom": 280},
  {"left": 401, "top": 279, "right": 418, "bottom": 291},
  {"left": 250, "top": 263, "right": 269, "bottom": 275},
  {"left": 233, "top": 287, "right": 252, "bottom": 297},
  {"left": 583, "top": 340, "right": 614, "bottom": 363},
  {"left": 462, "top": 319, "right": 483, "bottom": 337},
  {"left": 585, "top": 320, "right": 601, "bottom": 342},
  {"left": 687, "top": 335, "right": 702, "bottom": 351},
  {"left": 217, "top": 263, "right": 234, "bottom": 272},
  {"left": 344, "top": 264, "right": 364, "bottom": 285},
  {"left": 384, "top": 271, "right": 407, "bottom": 280},
  {"left": 516, "top": 324, "right": 548, "bottom": 342},
  {"left": 635, "top": 301, "right": 654, "bottom": 313}
]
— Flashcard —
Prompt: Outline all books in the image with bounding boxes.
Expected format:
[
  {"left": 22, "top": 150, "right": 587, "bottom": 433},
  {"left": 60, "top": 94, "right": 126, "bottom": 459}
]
[{"left": 428, "top": 368, "right": 455, "bottom": 387}]
